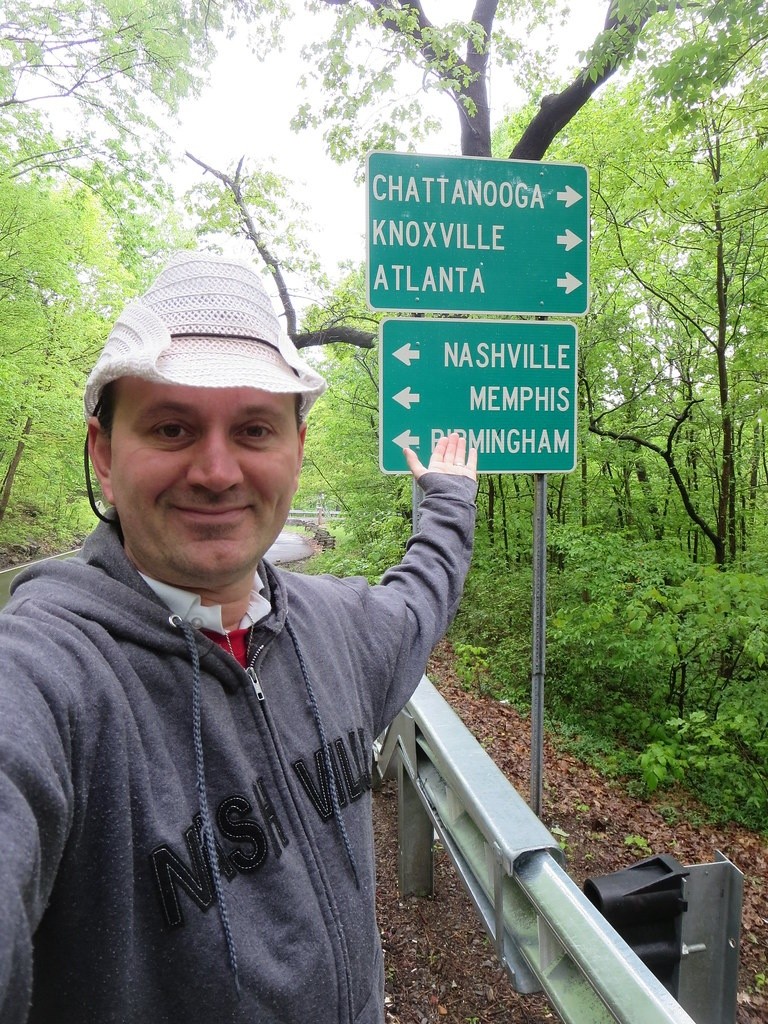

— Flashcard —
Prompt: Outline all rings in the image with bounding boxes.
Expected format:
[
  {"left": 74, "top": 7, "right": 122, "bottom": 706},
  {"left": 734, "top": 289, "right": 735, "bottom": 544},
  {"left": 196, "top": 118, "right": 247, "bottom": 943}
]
[{"left": 454, "top": 462, "right": 465, "bottom": 468}]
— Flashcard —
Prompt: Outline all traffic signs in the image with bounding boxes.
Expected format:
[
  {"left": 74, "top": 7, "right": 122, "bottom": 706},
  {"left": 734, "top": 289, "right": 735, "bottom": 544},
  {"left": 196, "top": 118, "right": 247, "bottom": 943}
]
[
  {"left": 378, "top": 317, "right": 577, "bottom": 475},
  {"left": 365, "top": 152, "right": 591, "bottom": 317}
]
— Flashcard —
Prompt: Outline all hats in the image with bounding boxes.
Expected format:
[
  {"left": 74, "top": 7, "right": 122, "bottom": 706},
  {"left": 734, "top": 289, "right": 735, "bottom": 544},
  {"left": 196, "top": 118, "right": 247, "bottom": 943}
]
[{"left": 83, "top": 249, "right": 327, "bottom": 524}]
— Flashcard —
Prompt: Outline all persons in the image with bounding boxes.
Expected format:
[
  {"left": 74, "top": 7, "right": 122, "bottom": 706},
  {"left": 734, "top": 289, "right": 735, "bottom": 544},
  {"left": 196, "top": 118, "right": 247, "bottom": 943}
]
[{"left": 0, "top": 251, "right": 478, "bottom": 1024}]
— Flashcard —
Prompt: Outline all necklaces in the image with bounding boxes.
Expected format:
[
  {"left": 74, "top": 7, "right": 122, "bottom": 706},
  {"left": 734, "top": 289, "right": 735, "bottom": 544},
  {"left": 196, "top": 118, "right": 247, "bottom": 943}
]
[{"left": 220, "top": 614, "right": 255, "bottom": 664}]
